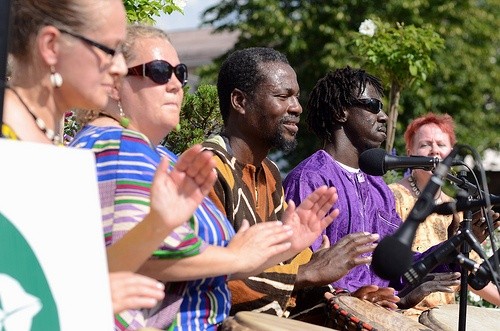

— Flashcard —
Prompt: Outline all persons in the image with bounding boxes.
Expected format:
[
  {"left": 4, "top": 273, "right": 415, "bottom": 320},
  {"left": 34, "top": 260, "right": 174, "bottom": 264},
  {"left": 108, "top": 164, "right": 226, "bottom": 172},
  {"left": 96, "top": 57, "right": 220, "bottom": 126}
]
[
  {"left": 198, "top": 47, "right": 401, "bottom": 319},
  {"left": 283, "top": 65, "right": 500, "bottom": 310},
  {"left": 0, "top": 0, "right": 217, "bottom": 316},
  {"left": 67, "top": 26, "right": 339, "bottom": 331},
  {"left": 387, "top": 112, "right": 500, "bottom": 322}
]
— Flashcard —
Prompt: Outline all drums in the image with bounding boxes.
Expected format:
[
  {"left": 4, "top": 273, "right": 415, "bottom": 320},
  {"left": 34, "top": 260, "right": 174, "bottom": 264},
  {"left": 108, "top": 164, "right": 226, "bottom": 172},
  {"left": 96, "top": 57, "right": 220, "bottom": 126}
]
[
  {"left": 419, "top": 301, "right": 500, "bottom": 331},
  {"left": 220, "top": 310, "right": 338, "bottom": 331},
  {"left": 327, "top": 294, "right": 437, "bottom": 330}
]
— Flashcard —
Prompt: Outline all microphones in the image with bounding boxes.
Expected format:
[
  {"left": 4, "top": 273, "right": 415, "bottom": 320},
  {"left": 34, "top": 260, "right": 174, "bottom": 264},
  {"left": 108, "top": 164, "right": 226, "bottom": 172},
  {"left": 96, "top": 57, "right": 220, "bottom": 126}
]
[{"left": 358, "top": 143, "right": 500, "bottom": 291}]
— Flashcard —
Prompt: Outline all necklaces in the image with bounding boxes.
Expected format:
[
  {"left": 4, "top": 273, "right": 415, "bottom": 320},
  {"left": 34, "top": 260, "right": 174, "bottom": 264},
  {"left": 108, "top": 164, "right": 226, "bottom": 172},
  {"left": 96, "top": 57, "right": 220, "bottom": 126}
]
[
  {"left": 5, "top": 85, "right": 65, "bottom": 147},
  {"left": 98, "top": 112, "right": 128, "bottom": 130},
  {"left": 408, "top": 176, "right": 442, "bottom": 205}
]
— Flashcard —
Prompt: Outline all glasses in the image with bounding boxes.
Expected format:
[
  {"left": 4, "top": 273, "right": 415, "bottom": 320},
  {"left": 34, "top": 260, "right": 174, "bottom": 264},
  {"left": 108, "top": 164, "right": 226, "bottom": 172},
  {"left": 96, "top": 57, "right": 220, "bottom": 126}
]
[
  {"left": 347, "top": 98, "right": 383, "bottom": 114},
  {"left": 128, "top": 59, "right": 188, "bottom": 89},
  {"left": 59, "top": 28, "right": 129, "bottom": 58}
]
